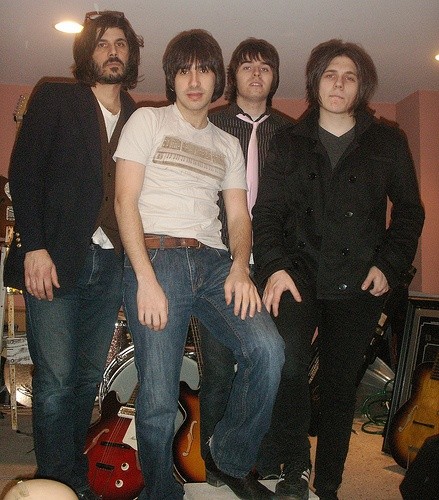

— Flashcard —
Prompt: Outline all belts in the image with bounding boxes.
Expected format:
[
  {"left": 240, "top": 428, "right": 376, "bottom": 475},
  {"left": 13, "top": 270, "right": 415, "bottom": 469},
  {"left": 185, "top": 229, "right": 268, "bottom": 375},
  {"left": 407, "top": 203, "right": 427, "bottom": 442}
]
[{"left": 145, "top": 234, "right": 206, "bottom": 249}]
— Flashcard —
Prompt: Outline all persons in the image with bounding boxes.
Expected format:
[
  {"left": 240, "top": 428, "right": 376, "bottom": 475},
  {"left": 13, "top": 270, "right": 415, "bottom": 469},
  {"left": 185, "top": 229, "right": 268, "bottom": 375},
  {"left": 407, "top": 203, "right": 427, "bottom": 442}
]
[
  {"left": 190, "top": 37, "right": 296, "bottom": 486},
  {"left": 3, "top": 13, "right": 139, "bottom": 500},
  {"left": 250, "top": 41, "right": 425, "bottom": 500},
  {"left": 111, "top": 29, "right": 285, "bottom": 500}
]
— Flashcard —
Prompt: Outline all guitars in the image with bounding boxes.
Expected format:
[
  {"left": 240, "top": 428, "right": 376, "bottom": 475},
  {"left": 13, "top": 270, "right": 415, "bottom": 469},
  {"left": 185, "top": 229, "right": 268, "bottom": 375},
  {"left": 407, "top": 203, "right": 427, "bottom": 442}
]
[
  {"left": 78, "top": 380, "right": 148, "bottom": 500},
  {"left": 171, "top": 314, "right": 206, "bottom": 484},
  {"left": 388, "top": 346, "right": 439, "bottom": 471}
]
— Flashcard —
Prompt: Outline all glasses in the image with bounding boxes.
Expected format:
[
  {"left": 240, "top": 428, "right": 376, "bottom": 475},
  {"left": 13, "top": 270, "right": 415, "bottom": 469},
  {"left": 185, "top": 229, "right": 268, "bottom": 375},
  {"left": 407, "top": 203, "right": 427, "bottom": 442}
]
[{"left": 85, "top": 10, "right": 124, "bottom": 25}]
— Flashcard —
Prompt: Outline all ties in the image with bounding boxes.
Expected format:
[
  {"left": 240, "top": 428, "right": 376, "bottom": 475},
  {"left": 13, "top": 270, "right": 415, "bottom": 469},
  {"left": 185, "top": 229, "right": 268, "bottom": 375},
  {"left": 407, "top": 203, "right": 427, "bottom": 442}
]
[{"left": 235, "top": 113, "right": 270, "bottom": 221}]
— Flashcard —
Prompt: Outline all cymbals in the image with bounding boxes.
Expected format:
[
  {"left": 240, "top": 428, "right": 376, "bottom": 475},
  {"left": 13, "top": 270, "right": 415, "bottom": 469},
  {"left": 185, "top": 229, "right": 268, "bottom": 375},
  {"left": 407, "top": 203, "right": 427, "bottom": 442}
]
[{"left": 3, "top": 341, "right": 36, "bottom": 407}]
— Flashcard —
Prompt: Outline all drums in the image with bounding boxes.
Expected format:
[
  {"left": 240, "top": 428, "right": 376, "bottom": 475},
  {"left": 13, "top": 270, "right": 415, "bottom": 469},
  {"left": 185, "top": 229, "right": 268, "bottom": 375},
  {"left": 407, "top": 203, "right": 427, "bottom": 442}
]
[
  {"left": 98, "top": 343, "right": 201, "bottom": 412},
  {"left": 101, "top": 320, "right": 132, "bottom": 372}
]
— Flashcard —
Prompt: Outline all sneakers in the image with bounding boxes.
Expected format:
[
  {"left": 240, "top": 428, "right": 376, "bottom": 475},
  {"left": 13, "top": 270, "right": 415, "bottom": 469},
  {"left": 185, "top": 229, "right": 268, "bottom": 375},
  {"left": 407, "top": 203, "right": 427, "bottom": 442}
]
[{"left": 275, "top": 462, "right": 312, "bottom": 500}]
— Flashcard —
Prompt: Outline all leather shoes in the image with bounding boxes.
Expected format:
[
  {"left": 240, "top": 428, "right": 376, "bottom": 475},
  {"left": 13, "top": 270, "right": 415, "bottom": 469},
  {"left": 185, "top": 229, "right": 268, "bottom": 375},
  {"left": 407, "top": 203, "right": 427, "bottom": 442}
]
[{"left": 205, "top": 450, "right": 278, "bottom": 500}]
